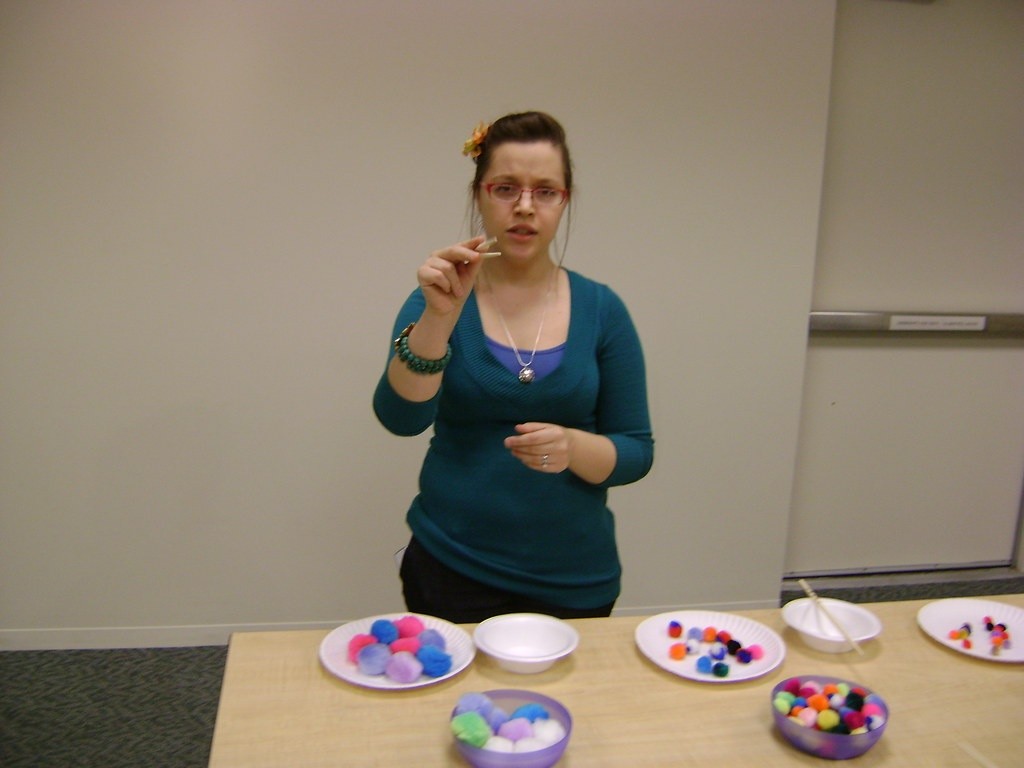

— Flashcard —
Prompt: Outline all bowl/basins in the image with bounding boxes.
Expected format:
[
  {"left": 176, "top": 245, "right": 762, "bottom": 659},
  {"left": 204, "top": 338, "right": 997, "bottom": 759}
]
[
  {"left": 780, "top": 597, "right": 882, "bottom": 654},
  {"left": 771, "top": 675, "right": 890, "bottom": 760},
  {"left": 473, "top": 612, "right": 579, "bottom": 675},
  {"left": 449, "top": 688, "right": 573, "bottom": 768}
]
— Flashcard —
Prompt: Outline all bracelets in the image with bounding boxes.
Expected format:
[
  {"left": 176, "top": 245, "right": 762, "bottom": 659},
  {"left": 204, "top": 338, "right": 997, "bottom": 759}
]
[{"left": 394, "top": 322, "right": 452, "bottom": 375}]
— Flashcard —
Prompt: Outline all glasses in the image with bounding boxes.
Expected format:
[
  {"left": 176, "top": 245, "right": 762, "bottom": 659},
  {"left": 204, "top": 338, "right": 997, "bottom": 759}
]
[{"left": 481, "top": 181, "right": 568, "bottom": 207}]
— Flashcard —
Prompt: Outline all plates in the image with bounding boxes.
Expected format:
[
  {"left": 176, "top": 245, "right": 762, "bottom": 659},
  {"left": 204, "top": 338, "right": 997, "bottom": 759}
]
[
  {"left": 917, "top": 598, "right": 1024, "bottom": 663},
  {"left": 318, "top": 612, "right": 476, "bottom": 690},
  {"left": 634, "top": 610, "right": 788, "bottom": 684}
]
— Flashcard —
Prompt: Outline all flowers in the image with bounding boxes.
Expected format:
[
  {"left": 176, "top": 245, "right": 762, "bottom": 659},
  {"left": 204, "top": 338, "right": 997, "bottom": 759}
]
[{"left": 460, "top": 121, "right": 490, "bottom": 163}]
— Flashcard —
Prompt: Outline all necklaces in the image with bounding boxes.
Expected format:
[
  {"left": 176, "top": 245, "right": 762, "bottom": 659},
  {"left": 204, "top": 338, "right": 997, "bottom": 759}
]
[{"left": 482, "top": 265, "right": 556, "bottom": 383}]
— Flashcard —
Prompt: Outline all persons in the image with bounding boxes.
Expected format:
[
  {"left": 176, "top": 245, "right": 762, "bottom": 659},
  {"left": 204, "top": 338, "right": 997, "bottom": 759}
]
[{"left": 372, "top": 110, "right": 654, "bottom": 624}]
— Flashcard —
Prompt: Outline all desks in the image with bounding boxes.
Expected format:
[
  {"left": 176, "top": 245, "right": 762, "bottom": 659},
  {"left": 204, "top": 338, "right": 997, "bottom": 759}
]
[{"left": 207, "top": 592, "right": 1024, "bottom": 768}]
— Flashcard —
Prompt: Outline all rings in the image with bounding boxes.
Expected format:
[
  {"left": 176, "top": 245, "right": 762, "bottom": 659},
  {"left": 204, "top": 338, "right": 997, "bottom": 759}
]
[{"left": 543, "top": 454, "right": 547, "bottom": 469}]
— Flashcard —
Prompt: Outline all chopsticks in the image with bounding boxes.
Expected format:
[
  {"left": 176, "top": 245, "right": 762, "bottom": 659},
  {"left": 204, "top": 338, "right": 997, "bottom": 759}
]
[{"left": 473, "top": 236, "right": 502, "bottom": 258}]
